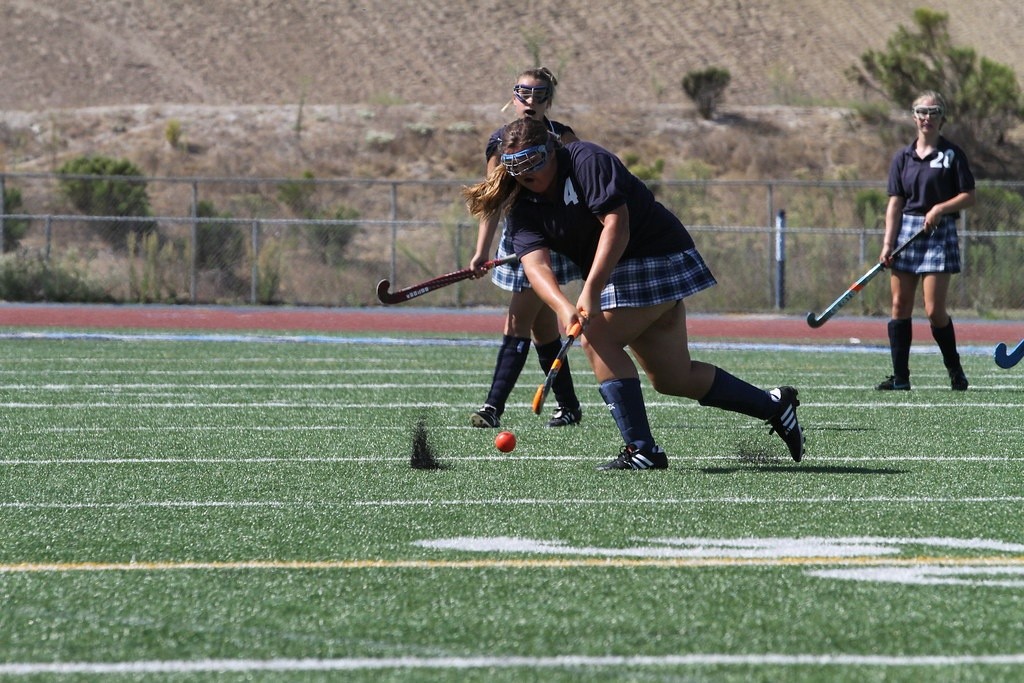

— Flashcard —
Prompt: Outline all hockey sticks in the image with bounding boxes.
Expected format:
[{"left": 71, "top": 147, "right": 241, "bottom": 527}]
[
  {"left": 377, "top": 253, "right": 515, "bottom": 303},
  {"left": 805, "top": 225, "right": 934, "bottom": 326},
  {"left": 992, "top": 336, "right": 1024, "bottom": 367},
  {"left": 533, "top": 310, "right": 587, "bottom": 413}
]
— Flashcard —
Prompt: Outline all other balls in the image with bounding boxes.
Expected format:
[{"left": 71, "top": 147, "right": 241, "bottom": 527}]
[{"left": 496, "top": 432, "right": 516, "bottom": 450}]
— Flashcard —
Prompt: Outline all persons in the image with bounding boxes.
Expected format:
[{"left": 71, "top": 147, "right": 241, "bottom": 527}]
[
  {"left": 875, "top": 90, "right": 977, "bottom": 393},
  {"left": 470, "top": 67, "right": 582, "bottom": 428},
  {"left": 459, "top": 116, "right": 808, "bottom": 472}
]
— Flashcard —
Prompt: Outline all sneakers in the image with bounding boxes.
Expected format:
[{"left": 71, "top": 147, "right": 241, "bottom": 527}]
[
  {"left": 875, "top": 374, "right": 910, "bottom": 390},
  {"left": 949, "top": 366, "right": 968, "bottom": 391},
  {"left": 764, "top": 386, "right": 806, "bottom": 462},
  {"left": 592, "top": 444, "right": 668, "bottom": 471},
  {"left": 469, "top": 404, "right": 500, "bottom": 428},
  {"left": 543, "top": 401, "right": 582, "bottom": 427}
]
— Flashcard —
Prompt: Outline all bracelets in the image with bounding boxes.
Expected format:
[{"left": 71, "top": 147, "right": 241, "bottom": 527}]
[{"left": 883, "top": 239, "right": 895, "bottom": 246}]
